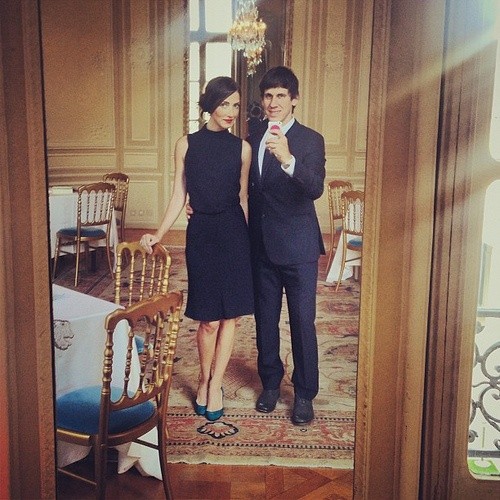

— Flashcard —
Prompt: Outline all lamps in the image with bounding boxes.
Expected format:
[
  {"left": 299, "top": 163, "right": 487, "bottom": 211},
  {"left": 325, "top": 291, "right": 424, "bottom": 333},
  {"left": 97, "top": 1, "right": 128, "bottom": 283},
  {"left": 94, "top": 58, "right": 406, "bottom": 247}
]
[{"left": 0, "top": 0, "right": 447, "bottom": 500}]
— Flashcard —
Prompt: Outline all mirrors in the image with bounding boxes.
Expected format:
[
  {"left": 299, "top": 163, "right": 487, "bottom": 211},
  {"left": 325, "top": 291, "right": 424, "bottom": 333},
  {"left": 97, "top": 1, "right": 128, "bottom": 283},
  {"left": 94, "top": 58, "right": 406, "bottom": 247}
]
[{"left": 230, "top": 0, "right": 267, "bottom": 78}]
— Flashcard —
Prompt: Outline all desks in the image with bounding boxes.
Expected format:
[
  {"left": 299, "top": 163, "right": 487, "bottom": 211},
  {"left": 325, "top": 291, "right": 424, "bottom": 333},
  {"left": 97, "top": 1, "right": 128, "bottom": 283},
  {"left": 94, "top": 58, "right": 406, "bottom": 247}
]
[
  {"left": 48, "top": 188, "right": 119, "bottom": 273},
  {"left": 52, "top": 283, "right": 164, "bottom": 481}
]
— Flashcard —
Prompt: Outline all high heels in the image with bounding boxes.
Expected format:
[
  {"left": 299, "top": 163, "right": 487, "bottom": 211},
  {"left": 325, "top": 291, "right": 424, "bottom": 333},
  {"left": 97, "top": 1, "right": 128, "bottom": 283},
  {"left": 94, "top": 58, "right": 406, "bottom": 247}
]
[
  {"left": 194, "top": 399, "right": 207, "bottom": 416},
  {"left": 206, "top": 388, "right": 223, "bottom": 420}
]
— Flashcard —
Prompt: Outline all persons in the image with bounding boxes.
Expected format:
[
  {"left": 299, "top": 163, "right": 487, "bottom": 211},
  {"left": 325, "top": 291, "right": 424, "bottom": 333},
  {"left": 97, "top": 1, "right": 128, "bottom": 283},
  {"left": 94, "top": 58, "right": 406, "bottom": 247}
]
[
  {"left": 186, "top": 65, "right": 326, "bottom": 426},
  {"left": 138, "top": 76, "right": 252, "bottom": 421}
]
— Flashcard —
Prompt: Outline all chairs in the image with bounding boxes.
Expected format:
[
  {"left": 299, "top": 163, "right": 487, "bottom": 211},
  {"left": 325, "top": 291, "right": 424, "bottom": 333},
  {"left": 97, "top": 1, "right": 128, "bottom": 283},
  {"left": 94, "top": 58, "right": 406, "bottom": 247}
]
[
  {"left": 56, "top": 241, "right": 188, "bottom": 500},
  {"left": 51, "top": 181, "right": 115, "bottom": 287},
  {"left": 102, "top": 172, "right": 130, "bottom": 266},
  {"left": 323, "top": 181, "right": 364, "bottom": 293}
]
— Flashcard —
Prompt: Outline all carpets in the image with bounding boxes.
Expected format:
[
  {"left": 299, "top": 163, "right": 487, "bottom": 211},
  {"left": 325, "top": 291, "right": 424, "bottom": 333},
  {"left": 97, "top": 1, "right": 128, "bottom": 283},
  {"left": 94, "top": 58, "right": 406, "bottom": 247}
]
[{"left": 51, "top": 245, "right": 361, "bottom": 470}]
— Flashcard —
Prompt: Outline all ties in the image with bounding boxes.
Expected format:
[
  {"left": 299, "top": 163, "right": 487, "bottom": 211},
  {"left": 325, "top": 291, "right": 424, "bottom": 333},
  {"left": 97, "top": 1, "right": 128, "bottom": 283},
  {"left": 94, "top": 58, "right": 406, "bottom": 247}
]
[{"left": 262, "top": 148, "right": 270, "bottom": 179}]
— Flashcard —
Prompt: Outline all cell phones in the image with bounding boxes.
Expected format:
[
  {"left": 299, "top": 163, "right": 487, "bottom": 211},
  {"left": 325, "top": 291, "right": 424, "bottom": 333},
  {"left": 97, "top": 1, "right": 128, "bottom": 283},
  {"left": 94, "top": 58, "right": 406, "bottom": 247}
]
[{"left": 268, "top": 124, "right": 280, "bottom": 150}]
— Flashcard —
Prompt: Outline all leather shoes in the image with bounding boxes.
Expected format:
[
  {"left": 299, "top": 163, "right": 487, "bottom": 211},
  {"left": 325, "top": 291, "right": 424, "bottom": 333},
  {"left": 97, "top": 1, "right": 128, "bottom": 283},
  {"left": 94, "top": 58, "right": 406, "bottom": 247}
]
[
  {"left": 256, "top": 385, "right": 280, "bottom": 413},
  {"left": 292, "top": 393, "right": 314, "bottom": 425}
]
